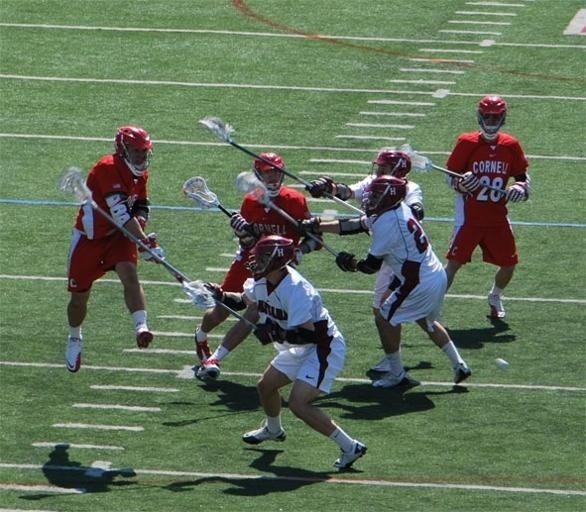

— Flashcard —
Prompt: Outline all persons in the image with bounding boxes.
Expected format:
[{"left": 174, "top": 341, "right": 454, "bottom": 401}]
[
  {"left": 194, "top": 151, "right": 323, "bottom": 382},
  {"left": 443, "top": 93, "right": 532, "bottom": 319},
  {"left": 201, "top": 235, "right": 368, "bottom": 470},
  {"left": 64, "top": 125, "right": 165, "bottom": 374},
  {"left": 307, "top": 150, "right": 424, "bottom": 372},
  {"left": 293, "top": 175, "right": 472, "bottom": 388}
]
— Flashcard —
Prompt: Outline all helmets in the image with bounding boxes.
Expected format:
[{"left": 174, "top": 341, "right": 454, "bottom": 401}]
[
  {"left": 372, "top": 150, "right": 412, "bottom": 179},
  {"left": 476, "top": 96, "right": 509, "bottom": 134},
  {"left": 115, "top": 125, "right": 153, "bottom": 177},
  {"left": 254, "top": 152, "right": 287, "bottom": 197},
  {"left": 364, "top": 176, "right": 408, "bottom": 216},
  {"left": 248, "top": 234, "right": 296, "bottom": 280}
]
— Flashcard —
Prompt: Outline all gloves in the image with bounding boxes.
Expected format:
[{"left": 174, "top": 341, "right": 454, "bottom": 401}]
[
  {"left": 502, "top": 180, "right": 529, "bottom": 204},
  {"left": 229, "top": 214, "right": 248, "bottom": 238},
  {"left": 304, "top": 176, "right": 335, "bottom": 198},
  {"left": 254, "top": 322, "right": 285, "bottom": 345},
  {"left": 451, "top": 171, "right": 480, "bottom": 196},
  {"left": 203, "top": 282, "right": 227, "bottom": 302},
  {"left": 336, "top": 249, "right": 358, "bottom": 273},
  {"left": 124, "top": 218, "right": 164, "bottom": 264}
]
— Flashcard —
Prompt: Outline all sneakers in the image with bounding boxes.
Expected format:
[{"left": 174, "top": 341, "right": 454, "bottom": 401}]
[
  {"left": 332, "top": 442, "right": 367, "bottom": 470},
  {"left": 371, "top": 353, "right": 411, "bottom": 390},
  {"left": 193, "top": 325, "right": 223, "bottom": 382},
  {"left": 66, "top": 335, "right": 82, "bottom": 372},
  {"left": 136, "top": 328, "right": 154, "bottom": 350},
  {"left": 486, "top": 291, "right": 508, "bottom": 319},
  {"left": 452, "top": 364, "right": 473, "bottom": 384},
  {"left": 243, "top": 424, "right": 287, "bottom": 446}
]
